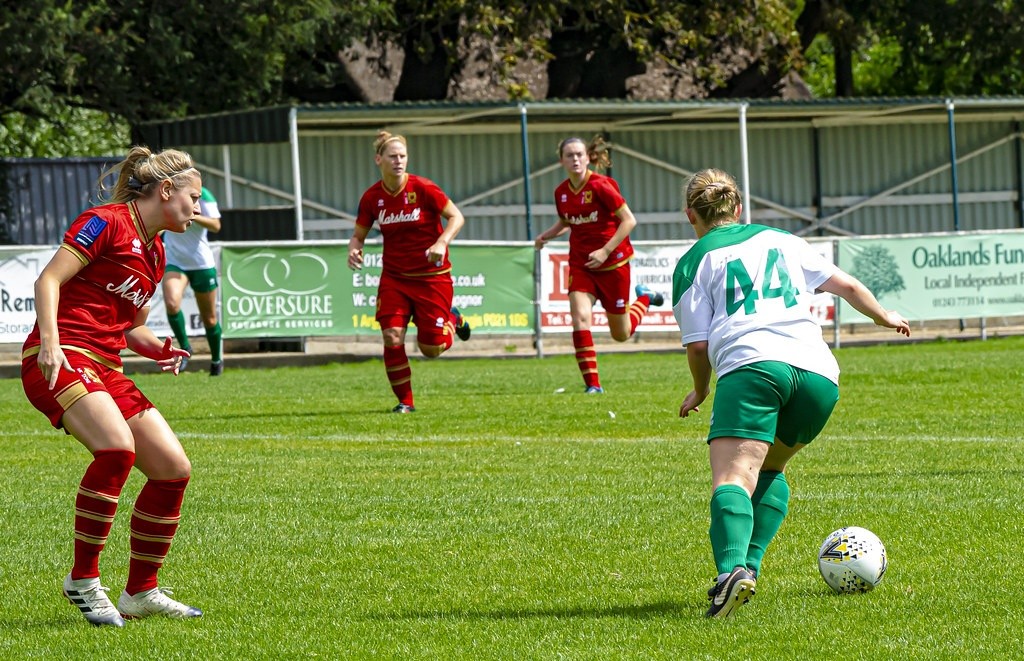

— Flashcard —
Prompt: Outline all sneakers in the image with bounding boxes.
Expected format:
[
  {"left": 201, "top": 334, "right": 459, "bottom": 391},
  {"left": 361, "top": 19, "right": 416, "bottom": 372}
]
[
  {"left": 451, "top": 307, "right": 470, "bottom": 340},
  {"left": 210, "top": 360, "right": 224, "bottom": 376},
  {"left": 636, "top": 285, "right": 663, "bottom": 305},
  {"left": 117, "top": 586, "right": 202, "bottom": 617},
  {"left": 179, "top": 347, "right": 192, "bottom": 372},
  {"left": 392, "top": 404, "right": 415, "bottom": 413},
  {"left": 584, "top": 387, "right": 603, "bottom": 393},
  {"left": 705, "top": 567, "right": 757, "bottom": 619},
  {"left": 63, "top": 571, "right": 126, "bottom": 628}
]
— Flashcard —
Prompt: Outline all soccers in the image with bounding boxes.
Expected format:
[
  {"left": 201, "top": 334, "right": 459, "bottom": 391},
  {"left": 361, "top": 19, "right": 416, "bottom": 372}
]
[{"left": 818, "top": 526, "right": 889, "bottom": 594}]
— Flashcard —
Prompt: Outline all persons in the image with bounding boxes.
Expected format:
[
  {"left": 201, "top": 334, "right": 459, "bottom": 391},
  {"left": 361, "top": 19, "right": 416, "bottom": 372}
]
[
  {"left": 22, "top": 148, "right": 203, "bottom": 626},
  {"left": 671, "top": 168, "right": 910, "bottom": 619},
  {"left": 535, "top": 137, "right": 664, "bottom": 395},
  {"left": 162, "top": 187, "right": 223, "bottom": 377},
  {"left": 347, "top": 131, "right": 470, "bottom": 413}
]
